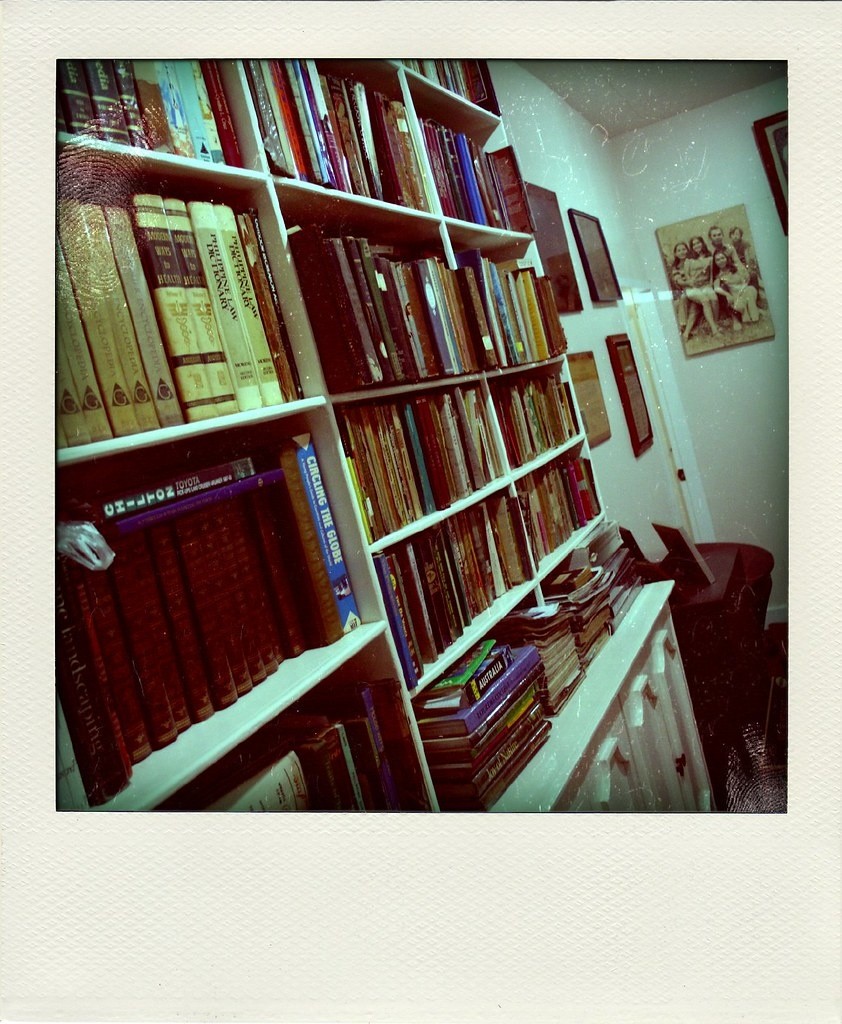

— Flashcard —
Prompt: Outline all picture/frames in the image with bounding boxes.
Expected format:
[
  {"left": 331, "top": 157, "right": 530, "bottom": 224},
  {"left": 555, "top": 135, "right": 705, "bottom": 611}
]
[
  {"left": 753, "top": 110, "right": 788, "bottom": 236},
  {"left": 652, "top": 521, "right": 715, "bottom": 585},
  {"left": 568, "top": 208, "right": 624, "bottom": 304}
]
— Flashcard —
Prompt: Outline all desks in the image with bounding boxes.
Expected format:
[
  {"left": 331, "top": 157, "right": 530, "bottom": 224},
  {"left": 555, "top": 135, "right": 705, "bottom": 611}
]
[
  {"left": 696, "top": 542, "right": 775, "bottom": 632},
  {"left": 657, "top": 546, "right": 769, "bottom": 811}
]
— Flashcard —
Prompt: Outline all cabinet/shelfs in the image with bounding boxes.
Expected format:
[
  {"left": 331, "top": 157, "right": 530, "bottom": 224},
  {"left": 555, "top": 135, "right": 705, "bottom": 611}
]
[{"left": 57, "top": 58, "right": 716, "bottom": 811}]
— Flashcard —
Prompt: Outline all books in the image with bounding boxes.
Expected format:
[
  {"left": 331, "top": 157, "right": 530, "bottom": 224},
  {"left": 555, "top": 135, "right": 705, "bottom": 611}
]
[{"left": 56, "top": 58, "right": 643, "bottom": 813}]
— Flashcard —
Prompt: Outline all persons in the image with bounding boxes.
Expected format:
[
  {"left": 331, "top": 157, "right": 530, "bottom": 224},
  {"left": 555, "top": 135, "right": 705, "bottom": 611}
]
[{"left": 671, "top": 225, "right": 768, "bottom": 342}]
